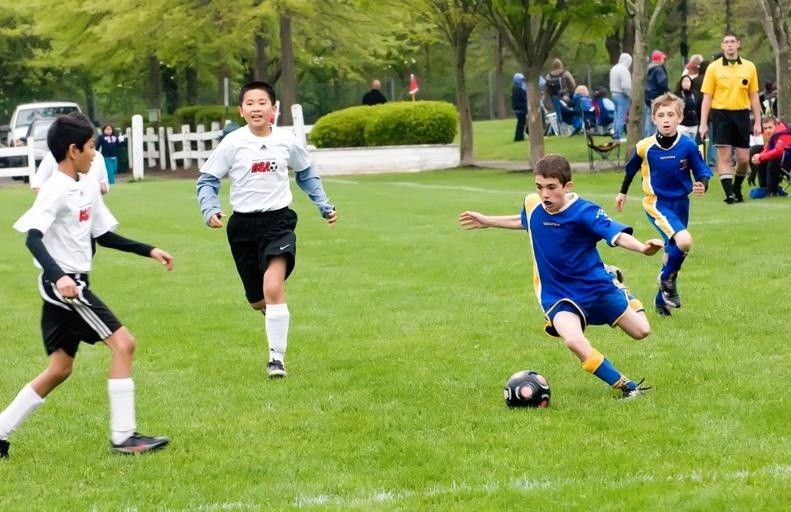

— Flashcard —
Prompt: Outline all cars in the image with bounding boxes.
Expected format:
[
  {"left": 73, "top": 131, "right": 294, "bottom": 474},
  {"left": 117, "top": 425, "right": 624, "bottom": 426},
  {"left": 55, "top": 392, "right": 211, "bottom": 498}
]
[{"left": 21, "top": 117, "right": 99, "bottom": 184}]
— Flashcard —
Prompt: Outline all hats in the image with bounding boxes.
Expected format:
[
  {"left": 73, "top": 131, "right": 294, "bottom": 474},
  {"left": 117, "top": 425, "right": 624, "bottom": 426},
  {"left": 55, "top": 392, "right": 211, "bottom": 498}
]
[{"left": 651, "top": 51, "right": 666, "bottom": 61}]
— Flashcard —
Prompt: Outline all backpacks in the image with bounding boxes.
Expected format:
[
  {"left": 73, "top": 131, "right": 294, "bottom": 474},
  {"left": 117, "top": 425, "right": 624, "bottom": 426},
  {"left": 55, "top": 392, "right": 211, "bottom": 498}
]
[{"left": 545, "top": 72, "right": 564, "bottom": 94}]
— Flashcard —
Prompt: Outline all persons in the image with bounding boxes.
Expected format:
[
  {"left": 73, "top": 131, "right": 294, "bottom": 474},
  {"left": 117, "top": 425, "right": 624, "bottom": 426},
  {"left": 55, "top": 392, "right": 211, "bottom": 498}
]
[
  {"left": 753, "top": 115, "right": 791, "bottom": 198},
  {"left": 696, "top": 31, "right": 765, "bottom": 205},
  {"left": 30, "top": 112, "right": 109, "bottom": 258},
  {"left": 614, "top": 92, "right": 715, "bottom": 316},
  {"left": 197, "top": 80, "right": 339, "bottom": 377},
  {"left": 1, "top": 113, "right": 177, "bottom": 459},
  {"left": 97, "top": 121, "right": 126, "bottom": 187},
  {"left": 457, "top": 152, "right": 665, "bottom": 402},
  {"left": 508, "top": 51, "right": 778, "bottom": 166},
  {"left": 364, "top": 79, "right": 387, "bottom": 105}
]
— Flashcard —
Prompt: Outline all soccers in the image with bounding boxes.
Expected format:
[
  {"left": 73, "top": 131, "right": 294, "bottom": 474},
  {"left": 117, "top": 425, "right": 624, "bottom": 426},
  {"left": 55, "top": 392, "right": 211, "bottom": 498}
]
[{"left": 503, "top": 370, "right": 550, "bottom": 408}]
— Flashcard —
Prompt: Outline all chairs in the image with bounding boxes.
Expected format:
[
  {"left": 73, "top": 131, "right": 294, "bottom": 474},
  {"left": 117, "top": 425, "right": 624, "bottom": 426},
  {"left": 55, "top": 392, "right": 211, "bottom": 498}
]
[
  {"left": 579, "top": 97, "right": 622, "bottom": 172},
  {"left": 769, "top": 163, "right": 791, "bottom": 195}
]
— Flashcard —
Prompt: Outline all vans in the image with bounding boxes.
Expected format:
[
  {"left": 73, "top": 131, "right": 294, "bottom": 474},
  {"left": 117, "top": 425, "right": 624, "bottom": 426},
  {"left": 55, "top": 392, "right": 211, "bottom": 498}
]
[{"left": 0, "top": 102, "right": 85, "bottom": 180}]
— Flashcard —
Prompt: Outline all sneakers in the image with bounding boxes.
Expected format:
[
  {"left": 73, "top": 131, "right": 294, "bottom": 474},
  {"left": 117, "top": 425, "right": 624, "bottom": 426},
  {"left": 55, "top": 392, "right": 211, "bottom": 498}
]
[
  {"left": 267, "top": 359, "right": 286, "bottom": 377},
  {"left": 111, "top": 433, "right": 170, "bottom": 454},
  {"left": 605, "top": 263, "right": 623, "bottom": 281},
  {"left": 623, "top": 387, "right": 641, "bottom": 401},
  {"left": 655, "top": 274, "right": 679, "bottom": 316},
  {"left": 724, "top": 185, "right": 743, "bottom": 203}
]
